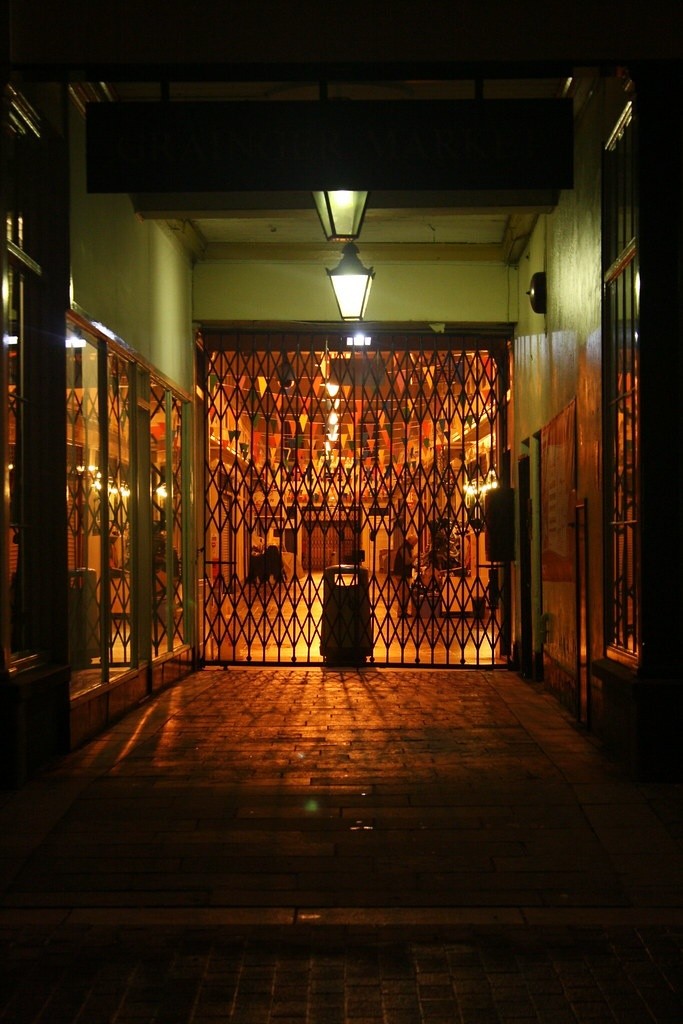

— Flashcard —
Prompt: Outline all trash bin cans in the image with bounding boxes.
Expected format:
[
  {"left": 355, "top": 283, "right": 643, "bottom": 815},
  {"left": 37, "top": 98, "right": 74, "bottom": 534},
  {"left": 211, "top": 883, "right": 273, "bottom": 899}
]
[
  {"left": 321, "top": 563, "right": 374, "bottom": 664},
  {"left": 67, "top": 568, "right": 99, "bottom": 662}
]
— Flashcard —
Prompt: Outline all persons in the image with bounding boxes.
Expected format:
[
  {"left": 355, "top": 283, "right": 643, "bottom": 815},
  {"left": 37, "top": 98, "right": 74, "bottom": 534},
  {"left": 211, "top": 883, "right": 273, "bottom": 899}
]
[{"left": 394, "top": 534, "right": 422, "bottom": 617}]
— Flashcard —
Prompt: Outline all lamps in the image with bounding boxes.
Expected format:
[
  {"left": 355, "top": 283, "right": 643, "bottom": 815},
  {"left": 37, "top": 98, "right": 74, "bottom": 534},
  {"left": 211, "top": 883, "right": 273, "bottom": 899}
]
[
  {"left": 326, "top": 242, "right": 377, "bottom": 323},
  {"left": 313, "top": 190, "right": 370, "bottom": 243}
]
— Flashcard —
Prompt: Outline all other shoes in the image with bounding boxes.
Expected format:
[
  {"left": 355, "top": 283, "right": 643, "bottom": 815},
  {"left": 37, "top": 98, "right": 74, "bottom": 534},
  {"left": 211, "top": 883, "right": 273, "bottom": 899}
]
[{"left": 398, "top": 612, "right": 412, "bottom": 618}]
